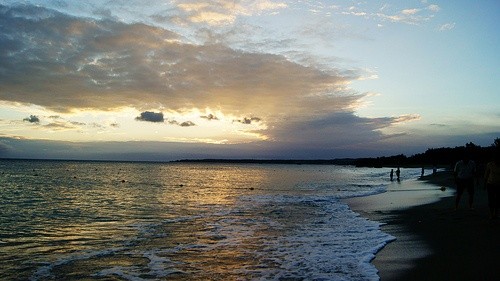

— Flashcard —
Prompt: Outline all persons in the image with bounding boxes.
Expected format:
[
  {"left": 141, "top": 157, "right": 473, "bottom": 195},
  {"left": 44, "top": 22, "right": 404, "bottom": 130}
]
[{"left": 386, "top": 165, "right": 439, "bottom": 181}]
